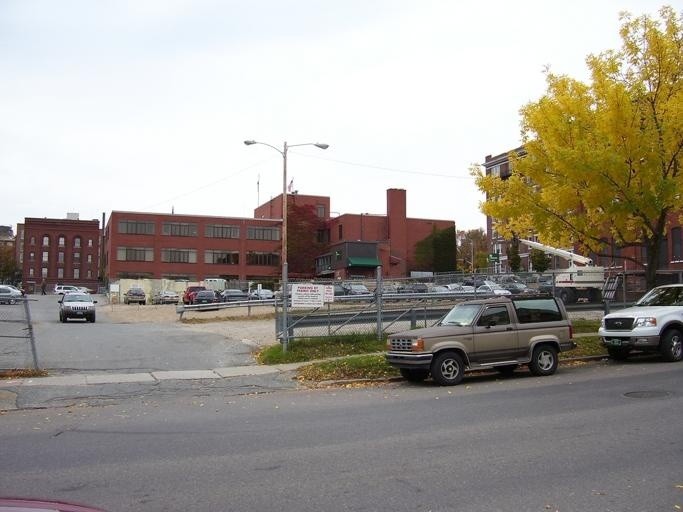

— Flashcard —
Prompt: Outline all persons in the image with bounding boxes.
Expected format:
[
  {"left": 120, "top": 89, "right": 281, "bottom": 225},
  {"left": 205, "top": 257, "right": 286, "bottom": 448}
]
[
  {"left": 178, "top": 289, "right": 195, "bottom": 305},
  {"left": 14, "top": 280, "right": 25, "bottom": 297},
  {"left": 39, "top": 277, "right": 47, "bottom": 295}
]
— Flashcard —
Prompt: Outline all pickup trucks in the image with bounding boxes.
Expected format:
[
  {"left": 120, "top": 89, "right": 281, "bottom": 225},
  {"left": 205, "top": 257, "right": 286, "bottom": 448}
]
[{"left": 182, "top": 286, "right": 208, "bottom": 305}]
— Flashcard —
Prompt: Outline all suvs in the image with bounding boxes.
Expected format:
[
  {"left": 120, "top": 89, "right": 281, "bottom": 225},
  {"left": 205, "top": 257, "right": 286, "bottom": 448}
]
[
  {"left": 193, "top": 291, "right": 220, "bottom": 311},
  {"left": 54, "top": 284, "right": 84, "bottom": 297},
  {"left": 384, "top": 296, "right": 579, "bottom": 387},
  {"left": 595, "top": 283, "right": 683, "bottom": 362},
  {"left": 58, "top": 292, "right": 99, "bottom": 324}
]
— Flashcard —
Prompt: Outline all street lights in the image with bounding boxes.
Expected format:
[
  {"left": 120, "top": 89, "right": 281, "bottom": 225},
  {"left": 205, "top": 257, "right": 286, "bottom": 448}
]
[
  {"left": 460, "top": 237, "right": 473, "bottom": 272},
  {"left": 242, "top": 138, "right": 332, "bottom": 353}
]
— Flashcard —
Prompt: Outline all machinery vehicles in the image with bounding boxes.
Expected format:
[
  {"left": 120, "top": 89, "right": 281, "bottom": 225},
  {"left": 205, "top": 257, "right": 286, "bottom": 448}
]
[{"left": 516, "top": 238, "right": 606, "bottom": 304}]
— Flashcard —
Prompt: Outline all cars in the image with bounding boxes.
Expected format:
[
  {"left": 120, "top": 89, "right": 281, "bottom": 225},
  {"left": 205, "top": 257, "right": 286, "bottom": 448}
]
[
  {"left": 0, "top": 494, "right": 110, "bottom": 511},
  {"left": 248, "top": 289, "right": 275, "bottom": 300},
  {"left": 273, "top": 264, "right": 551, "bottom": 309},
  {"left": 219, "top": 288, "right": 259, "bottom": 303},
  {"left": 79, "top": 287, "right": 97, "bottom": 294},
  {"left": 0, "top": 284, "right": 26, "bottom": 305},
  {"left": 123, "top": 287, "right": 146, "bottom": 306},
  {"left": 151, "top": 290, "right": 181, "bottom": 306}
]
